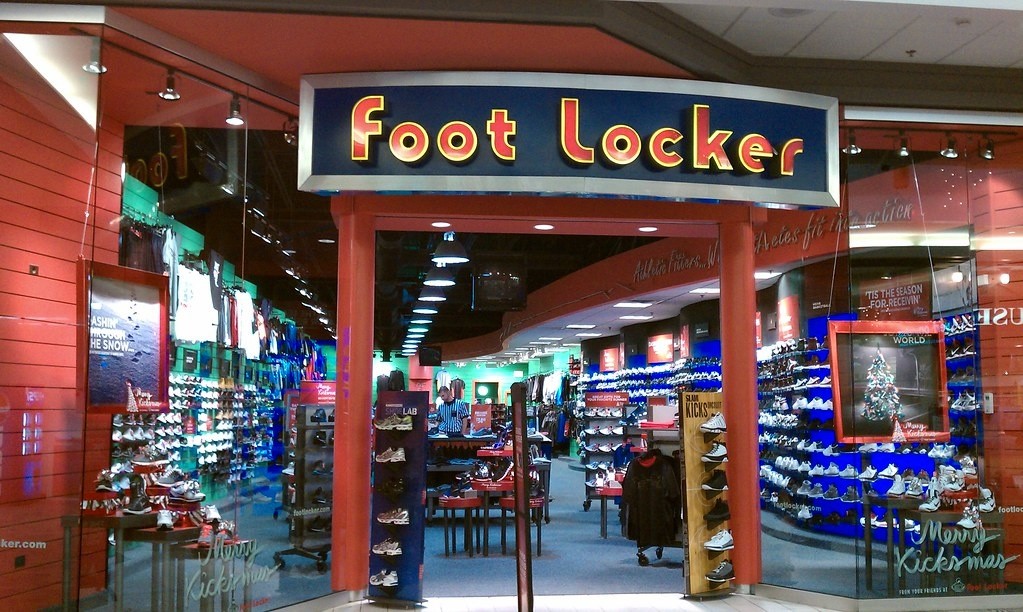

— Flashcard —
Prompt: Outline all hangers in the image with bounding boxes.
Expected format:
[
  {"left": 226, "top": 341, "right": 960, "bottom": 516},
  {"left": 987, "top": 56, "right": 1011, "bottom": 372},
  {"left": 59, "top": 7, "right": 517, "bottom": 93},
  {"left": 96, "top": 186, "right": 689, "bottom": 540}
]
[
  {"left": 125, "top": 207, "right": 177, "bottom": 234},
  {"left": 226, "top": 279, "right": 247, "bottom": 292}
]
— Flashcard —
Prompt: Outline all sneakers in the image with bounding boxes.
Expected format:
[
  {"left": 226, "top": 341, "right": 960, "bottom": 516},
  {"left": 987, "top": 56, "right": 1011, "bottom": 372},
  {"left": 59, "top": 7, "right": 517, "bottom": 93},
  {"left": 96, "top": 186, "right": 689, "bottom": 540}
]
[
  {"left": 426, "top": 335, "right": 998, "bottom": 582},
  {"left": 377, "top": 507, "right": 410, "bottom": 524},
  {"left": 372, "top": 538, "right": 403, "bottom": 555},
  {"left": 383, "top": 477, "right": 419, "bottom": 494},
  {"left": 376, "top": 446, "right": 406, "bottom": 462},
  {"left": 370, "top": 568, "right": 398, "bottom": 586},
  {"left": 373, "top": 413, "right": 413, "bottom": 431},
  {"left": 95, "top": 367, "right": 335, "bottom": 547}
]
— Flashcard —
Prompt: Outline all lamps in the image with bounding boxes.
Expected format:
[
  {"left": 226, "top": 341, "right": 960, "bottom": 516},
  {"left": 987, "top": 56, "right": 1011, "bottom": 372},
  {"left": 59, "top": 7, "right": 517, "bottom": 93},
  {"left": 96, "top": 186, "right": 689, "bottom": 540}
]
[
  {"left": 223, "top": 179, "right": 469, "bottom": 357},
  {"left": 224, "top": 93, "right": 245, "bottom": 126},
  {"left": 159, "top": 71, "right": 180, "bottom": 101},
  {"left": 280, "top": 117, "right": 299, "bottom": 145},
  {"left": 977, "top": 133, "right": 994, "bottom": 160},
  {"left": 897, "top": 130, "right": 910, "bottom": 157},
  {"left": 81, "top": 41, "right": 107, "bottom": 74},
  {"left": 940, "top": 131, "right": 958, "bottom": 158},
  {"left": 842, "top": 128, "right": 862, "bottom": 155}
]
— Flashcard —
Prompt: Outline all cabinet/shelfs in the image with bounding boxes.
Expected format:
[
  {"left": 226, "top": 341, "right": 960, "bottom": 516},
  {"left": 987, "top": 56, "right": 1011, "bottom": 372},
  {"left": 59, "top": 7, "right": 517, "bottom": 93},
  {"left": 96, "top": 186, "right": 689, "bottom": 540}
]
[{"left": 89, "top": 326, "right": 1002, "bottom": 611}]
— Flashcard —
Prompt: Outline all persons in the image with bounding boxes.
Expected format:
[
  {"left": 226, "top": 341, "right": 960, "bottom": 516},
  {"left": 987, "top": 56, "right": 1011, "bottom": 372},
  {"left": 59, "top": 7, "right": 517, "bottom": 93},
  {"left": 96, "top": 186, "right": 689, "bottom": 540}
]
[{"left": 434, "top": 386, "right": 470, "bottom": 435}]
